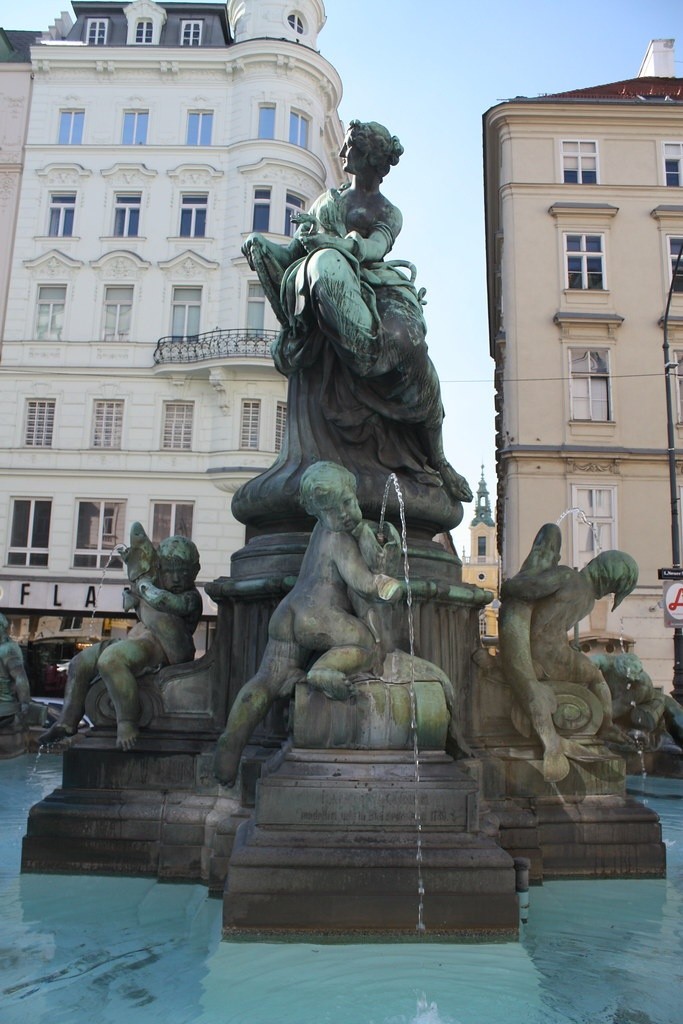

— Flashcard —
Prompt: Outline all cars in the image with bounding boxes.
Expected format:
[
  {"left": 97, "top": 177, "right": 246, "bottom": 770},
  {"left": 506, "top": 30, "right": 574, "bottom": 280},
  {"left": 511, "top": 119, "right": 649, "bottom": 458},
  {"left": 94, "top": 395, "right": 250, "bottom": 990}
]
[{"left": 57, "top": 659, "right": 71, "bottom": 676}]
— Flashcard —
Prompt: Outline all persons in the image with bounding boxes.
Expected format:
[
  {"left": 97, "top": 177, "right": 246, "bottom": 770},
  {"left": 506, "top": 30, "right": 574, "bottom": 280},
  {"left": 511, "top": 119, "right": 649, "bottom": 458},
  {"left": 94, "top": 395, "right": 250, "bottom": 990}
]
[
  {"left": 213, "top": 460, "right": 404, "bottom": 789},
  {"left": 0, "top": 614, "right": 30, "bottom": 723},
  {"left": 498, "top": 550, "right": 640, "bottom": 745},
  {"left": 241, "top": 119, "right": 474, "bottom": 503},
  {"left": 39, "top": 534, "right": 204, "bottom": 751}
]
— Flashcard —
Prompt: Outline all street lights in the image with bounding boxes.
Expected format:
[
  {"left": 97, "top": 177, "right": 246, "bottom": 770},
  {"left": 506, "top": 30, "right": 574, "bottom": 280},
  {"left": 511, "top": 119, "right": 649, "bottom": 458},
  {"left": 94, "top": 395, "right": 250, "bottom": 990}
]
[{"left": 664, "top": 361, "right": 683, "bottom": 701}]
